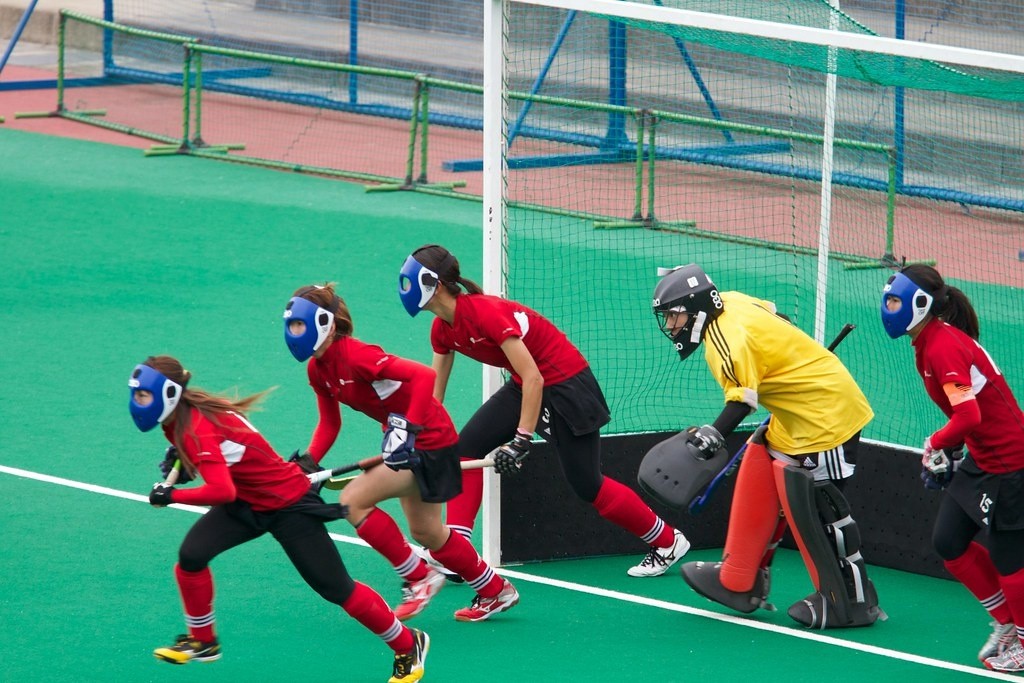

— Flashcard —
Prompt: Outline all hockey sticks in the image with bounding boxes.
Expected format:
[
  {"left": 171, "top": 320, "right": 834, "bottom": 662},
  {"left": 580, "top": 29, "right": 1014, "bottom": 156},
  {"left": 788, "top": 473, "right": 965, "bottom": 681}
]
[
  {"left": 305, "top": 455, "right": 384, "bottom": 483},
  {"left": 153, "top": 459, "right": 181, "bottom": 508},
  {"left": 688, "top": 322, "right": 856, "bottom": 516},
  {"left": 322, "top": 458, "right": 495, "bottom": 490}
]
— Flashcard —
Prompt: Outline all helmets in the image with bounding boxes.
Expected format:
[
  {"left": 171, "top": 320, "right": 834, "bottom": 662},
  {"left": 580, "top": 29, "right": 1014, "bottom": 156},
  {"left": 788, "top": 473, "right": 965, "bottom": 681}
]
[{"left": 652, "top": 264, "right": 723, "bottom": 361}]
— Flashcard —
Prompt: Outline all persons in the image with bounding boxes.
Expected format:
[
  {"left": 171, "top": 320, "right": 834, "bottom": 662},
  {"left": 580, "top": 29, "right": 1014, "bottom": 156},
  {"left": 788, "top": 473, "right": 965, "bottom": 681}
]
[
  {"left": 637, "top": 263, "right": 880, "bottom": 631},
  {"left": 398, "top": 245, "right": 691, "bottom": 583},
  {"left": 881, "top": 265, "right": 1024, "bottom": 673},
  {"left": 284, "top": 285, "right": 521, "bottom": 623},
  {"left": 128, "top": 356, "right": 430, "bottom": 683}
]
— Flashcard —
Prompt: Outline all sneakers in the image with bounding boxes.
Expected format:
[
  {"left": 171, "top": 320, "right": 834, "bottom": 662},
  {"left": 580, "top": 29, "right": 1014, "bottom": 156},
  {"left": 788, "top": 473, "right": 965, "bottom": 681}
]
[
  {"left": 388, "top": 628, "right": 430, "bottom": 683},
  {"left": 979, "top": 621, "right": 1017, "bottom": 661},
  {"left": 985, "top": 640, "right": 1024, "bottom": 671},
  {"left": 627, "top": 528, "right": 690, "bottom": 578},
  {"left": 407, "top": 544, "right": 465, "bottom": 582},
  {"left": 153, "top": 634, "right": 221, "bottom": 664},
  {"left": 393, "top": 565, "right": 446, "bottom": 620},
  {"left": 455, "top": 578, "right": 519, "bottom": 621}
]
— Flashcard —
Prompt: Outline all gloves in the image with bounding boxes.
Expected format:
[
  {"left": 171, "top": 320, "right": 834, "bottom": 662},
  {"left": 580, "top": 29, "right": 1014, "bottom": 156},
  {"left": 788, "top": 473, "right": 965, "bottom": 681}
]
[
  {"left": 149, "top": 482, "right": 175, "bottom": 507},
  {"left": 918, "top": 446, "right": 964, "bottom": 490},
  {"left": 493, "top": 438, "right": 532, "bottom": 475},
  {"left": 382, "top": 413, "right": 420, "bottom": 471},
  {"left": 289, "top": 450, "right": 327, "bottom": 493},
  {"left": 160, "top": 447, "right": 193, "bottom": 485}
]
